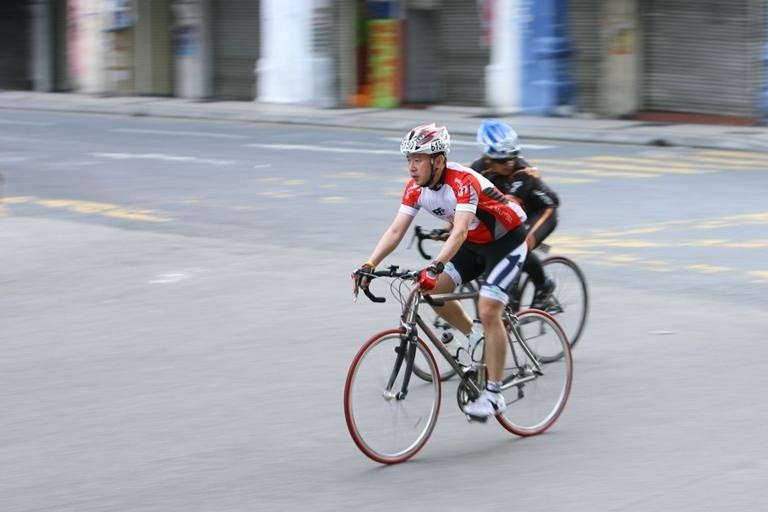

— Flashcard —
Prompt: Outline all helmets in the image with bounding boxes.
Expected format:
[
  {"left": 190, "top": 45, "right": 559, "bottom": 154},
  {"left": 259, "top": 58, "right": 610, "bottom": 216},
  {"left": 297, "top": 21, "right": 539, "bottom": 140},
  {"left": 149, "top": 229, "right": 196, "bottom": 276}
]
[
  {"left": 399, "top": 121, "right": 453, "bottom": 156},
  {"left": 477, "top": 116, "right": 522, "bottom": 161}
]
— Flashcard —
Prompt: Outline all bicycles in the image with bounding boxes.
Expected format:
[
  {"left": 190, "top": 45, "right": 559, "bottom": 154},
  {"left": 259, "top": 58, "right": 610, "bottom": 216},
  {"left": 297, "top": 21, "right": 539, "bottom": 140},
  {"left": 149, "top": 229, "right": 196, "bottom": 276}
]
[
  {"left": 400, "top": 226, "right": 587, "bottom": 382},
  {"left": 344, "top": 264, "right": 574, "bottom": 464}
]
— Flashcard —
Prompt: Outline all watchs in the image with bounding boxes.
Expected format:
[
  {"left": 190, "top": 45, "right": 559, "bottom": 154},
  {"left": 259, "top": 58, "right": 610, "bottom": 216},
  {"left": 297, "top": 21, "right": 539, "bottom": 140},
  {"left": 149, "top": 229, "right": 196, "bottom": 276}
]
[{"left": 431, "top": 259, "right": 447, "bottom": 272}]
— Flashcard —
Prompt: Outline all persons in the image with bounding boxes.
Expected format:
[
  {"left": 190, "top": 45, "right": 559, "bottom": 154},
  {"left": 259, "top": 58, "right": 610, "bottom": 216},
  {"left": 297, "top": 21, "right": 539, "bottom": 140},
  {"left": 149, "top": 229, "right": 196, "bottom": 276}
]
[
  {"left": 348, "top": 122, "right": 532, "bottom": 421},
  {"left": 428, "top": 118, "right": 560, "bottom": 316}
]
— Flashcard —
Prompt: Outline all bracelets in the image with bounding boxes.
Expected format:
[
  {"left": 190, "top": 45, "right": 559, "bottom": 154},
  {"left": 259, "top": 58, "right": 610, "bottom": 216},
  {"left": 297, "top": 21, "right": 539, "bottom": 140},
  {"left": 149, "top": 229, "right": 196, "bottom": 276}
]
[{"left": 369, "top": 261, "right": 376, "bottom": 268}]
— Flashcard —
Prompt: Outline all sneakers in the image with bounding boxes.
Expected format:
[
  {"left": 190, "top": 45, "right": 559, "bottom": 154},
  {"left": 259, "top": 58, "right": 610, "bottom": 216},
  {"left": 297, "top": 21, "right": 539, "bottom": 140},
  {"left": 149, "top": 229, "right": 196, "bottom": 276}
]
[
  {"left": 529, "top": 277, "right": 557, "bottom": 313},
  {"left": 462, "top": 386, "right": 508, "bottom": 420},
  {"left": 466, "top": 320, "right": 483, "bottom": 362}
]
